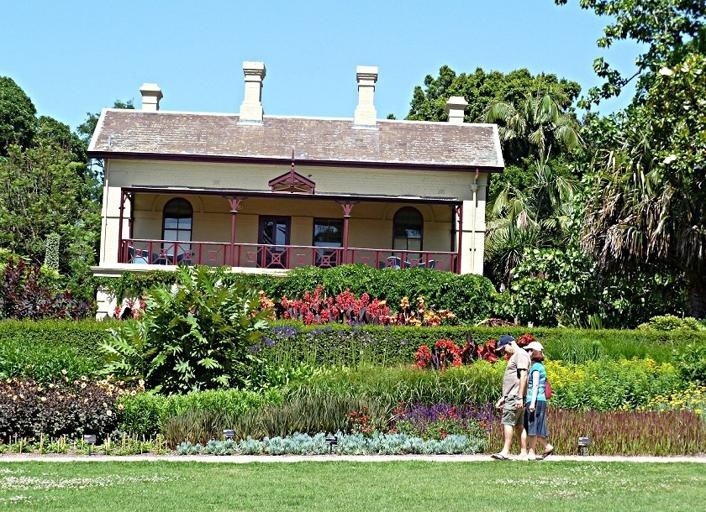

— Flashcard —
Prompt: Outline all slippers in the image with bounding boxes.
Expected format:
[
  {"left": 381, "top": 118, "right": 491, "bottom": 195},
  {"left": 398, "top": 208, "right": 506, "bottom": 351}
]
[{"left": 491, "top": 453, "right": 508, "bottom": 460}]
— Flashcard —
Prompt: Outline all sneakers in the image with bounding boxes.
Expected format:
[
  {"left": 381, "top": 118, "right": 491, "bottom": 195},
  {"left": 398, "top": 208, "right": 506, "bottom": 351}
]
[
  {"left": 542, "top": 446, "right": 555, "bottom": 458},
  {"left": 516, "top": 453, "right": 536, "bottom": 461}
]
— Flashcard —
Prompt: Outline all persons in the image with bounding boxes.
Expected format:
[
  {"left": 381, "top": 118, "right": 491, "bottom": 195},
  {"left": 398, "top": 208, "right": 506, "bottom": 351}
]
[
  {"left": 490, "top": 335, "right": 529, "bottom": 461},
  {"left": 118, "top": 297, "right": 134, "bottom": 320},
  {"left": 522, "top": 341, "right": 555, "bottom": 461}
]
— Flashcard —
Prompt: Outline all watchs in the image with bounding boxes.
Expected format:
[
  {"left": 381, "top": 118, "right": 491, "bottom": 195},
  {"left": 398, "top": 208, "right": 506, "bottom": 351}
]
[{"left": 516, "top": 396, "right": 523, "bottom": 400}]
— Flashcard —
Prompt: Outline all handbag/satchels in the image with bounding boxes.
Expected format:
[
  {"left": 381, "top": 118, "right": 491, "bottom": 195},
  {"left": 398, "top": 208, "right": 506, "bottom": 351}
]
[{"left": 545, "top": 383, "right": 552, "bottom": 398}]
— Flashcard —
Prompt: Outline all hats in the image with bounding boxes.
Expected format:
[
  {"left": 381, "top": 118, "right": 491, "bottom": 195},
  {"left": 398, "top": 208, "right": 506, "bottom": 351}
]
[
  {"left": 495, "top": 336, "right": 514, "bottom": 350},
  {"left": 523, "top": 342, "right": 545, "bottom": 350}
]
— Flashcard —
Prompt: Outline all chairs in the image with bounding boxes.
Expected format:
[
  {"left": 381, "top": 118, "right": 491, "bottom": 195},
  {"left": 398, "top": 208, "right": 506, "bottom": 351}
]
[
  {"left": 374, "top": 256, "right": 435, "bottom": 271},
  {"left": 124, "top": 245, "right": 194, "bottom": 266}
]
